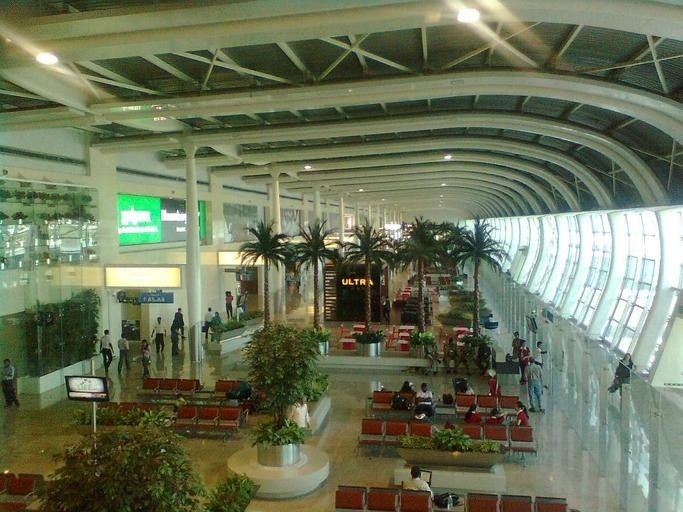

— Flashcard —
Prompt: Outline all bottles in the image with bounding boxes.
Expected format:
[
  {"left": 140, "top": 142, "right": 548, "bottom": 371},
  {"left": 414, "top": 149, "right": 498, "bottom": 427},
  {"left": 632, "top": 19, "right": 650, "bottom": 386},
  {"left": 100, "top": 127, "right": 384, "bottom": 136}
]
[{"left": 447, "top": 495, "right": 452, "bottom": 511}]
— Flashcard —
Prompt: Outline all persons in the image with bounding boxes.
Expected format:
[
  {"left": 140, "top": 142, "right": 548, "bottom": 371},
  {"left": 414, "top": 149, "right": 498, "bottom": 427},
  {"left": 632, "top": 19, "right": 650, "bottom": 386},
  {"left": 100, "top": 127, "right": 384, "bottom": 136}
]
[
  {"left": 402, "top": 466, "right": 434, "bottom": 512},
  {"left": 383, "top": 296, "right": 390, "bottom": 321},
  {"left": 401, "top": 331, "right": 545, "bottom": 427},
  {"left": 614, "top": 353, "right": 634, "bottom": 399},
  {"left": 100, "top": 289, "right": 257, "bottom": 413},
  {"left": 1, "top": 359, "right": 20, "bottom": 408},
  {"left": 506, "top": 269, "right": 511, "bottom": 276}
]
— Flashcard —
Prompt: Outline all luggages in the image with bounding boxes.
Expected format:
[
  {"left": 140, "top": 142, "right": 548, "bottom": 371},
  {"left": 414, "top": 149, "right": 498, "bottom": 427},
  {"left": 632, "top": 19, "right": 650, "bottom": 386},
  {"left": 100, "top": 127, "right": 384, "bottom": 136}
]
[{"left": 453, "top": 378, "right": 467, "bottom": 393}]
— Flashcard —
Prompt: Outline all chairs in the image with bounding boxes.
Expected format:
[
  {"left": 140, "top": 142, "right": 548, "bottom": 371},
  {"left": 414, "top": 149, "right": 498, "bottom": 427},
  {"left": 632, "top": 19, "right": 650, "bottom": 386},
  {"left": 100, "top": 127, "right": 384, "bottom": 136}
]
[
  {"left": 337, "top": 323, "right": 468, "bottom": 352},
  {"left": 336, "top": 391, "right": 567, "bottom": 512},
  {"left": 0, "top": 471, "right": 44, "bottom": 512},
  {"left": 393, "top": 275, "right": 453, "bottom": 306},
  {"left": 99, "top": 378, "right": 266, "bottom": 441}
]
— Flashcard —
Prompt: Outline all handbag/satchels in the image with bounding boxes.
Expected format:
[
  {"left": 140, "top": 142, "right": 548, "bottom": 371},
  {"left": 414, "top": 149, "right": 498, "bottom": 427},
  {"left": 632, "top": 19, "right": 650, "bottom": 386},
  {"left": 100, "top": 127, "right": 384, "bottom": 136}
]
[
  {"left": 415, "top": 404, "right": 432, "bottom": 417},
  {"left": 608, "top": 376, "right": 622, "bottom": 393},
  {"left": 443, "top": 394, "right": 453, "bottom": 404},
  {"left": 142, "top": 358, "right": 151, "bottom": 365}
]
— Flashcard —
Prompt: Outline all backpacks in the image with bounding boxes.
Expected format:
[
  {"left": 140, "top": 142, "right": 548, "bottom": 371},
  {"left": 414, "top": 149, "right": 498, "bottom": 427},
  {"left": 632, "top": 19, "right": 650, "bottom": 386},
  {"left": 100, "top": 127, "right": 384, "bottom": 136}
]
[{"left": 435, "top": 493, "right": 458, "bottom": 507}]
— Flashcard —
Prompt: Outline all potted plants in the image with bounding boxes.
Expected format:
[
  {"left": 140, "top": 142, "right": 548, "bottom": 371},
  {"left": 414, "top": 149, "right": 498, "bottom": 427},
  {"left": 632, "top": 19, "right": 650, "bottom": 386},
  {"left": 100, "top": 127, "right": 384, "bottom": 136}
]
[
  {"left": 210, "top": 321, "right": 247, "bottom": 341},
  {"left": 231, "top": 310, "right": 264, "bottom": 326},
  {"left": 73, "top": 408, "right": 176, "bottom": 435},
  {"left": 462, "top": 331, "right": 496, "bottom": 358},
  {"left": 249, "top": 417, "right": 305, "bottom": 467},
  {"left": 311, "top": 327, "right": 331, "bottom": 355}
]
